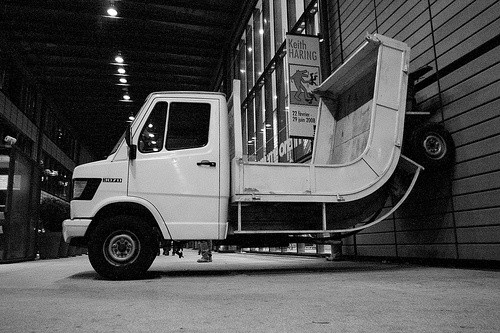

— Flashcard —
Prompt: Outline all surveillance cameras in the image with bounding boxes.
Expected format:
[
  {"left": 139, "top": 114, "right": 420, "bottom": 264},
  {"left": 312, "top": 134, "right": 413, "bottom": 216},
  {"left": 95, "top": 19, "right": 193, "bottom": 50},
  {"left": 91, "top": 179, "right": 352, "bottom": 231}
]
[{"left": 4, "top": 136, "right": 17, "bottom": 145}]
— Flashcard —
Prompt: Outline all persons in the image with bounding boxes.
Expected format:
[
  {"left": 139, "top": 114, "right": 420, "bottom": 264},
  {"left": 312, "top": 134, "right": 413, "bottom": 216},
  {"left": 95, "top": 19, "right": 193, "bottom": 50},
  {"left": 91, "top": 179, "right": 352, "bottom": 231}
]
[
  {"left": 325, "top": 234, "right": 344, "bottom": 262},
  {"left": 155, "top": 240, "right": 183, "bottom": 257},
  {"left": 198, "top": 240, "right": 213, "bottom": 263}
]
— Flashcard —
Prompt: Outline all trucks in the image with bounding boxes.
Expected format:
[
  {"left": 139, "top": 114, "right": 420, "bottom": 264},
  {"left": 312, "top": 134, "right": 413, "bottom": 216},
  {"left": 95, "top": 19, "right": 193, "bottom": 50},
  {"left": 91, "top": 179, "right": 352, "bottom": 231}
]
[{"left": 60, "top": 31, "right": 458, "bottom": 283}]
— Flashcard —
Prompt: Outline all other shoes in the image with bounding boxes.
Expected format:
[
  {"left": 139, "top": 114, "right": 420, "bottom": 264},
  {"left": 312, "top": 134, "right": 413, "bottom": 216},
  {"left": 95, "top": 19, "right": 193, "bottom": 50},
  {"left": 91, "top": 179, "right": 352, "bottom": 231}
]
[{"left": 197, "top": 258, "right": 212, "bottom": 262}]
